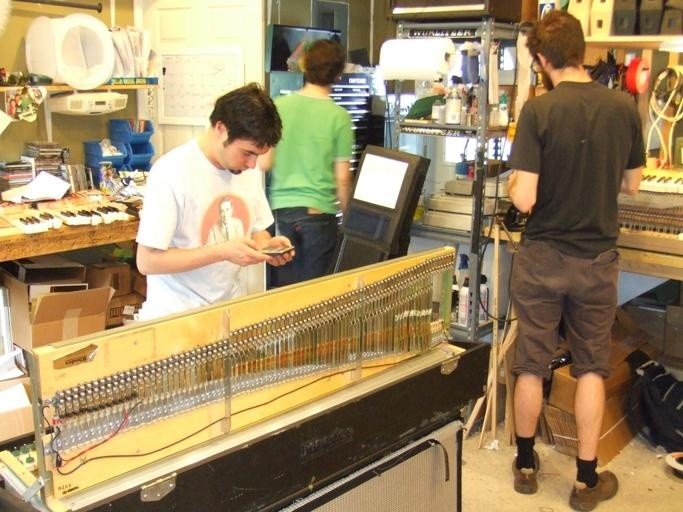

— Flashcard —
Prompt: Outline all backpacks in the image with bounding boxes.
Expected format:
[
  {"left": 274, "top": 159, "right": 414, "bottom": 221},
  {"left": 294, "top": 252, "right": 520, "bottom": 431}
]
[{"left": 626, "top": 359, "right": 683, "bottom": 454}]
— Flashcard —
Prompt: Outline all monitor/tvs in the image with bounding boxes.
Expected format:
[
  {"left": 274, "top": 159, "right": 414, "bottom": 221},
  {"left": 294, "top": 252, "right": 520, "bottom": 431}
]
[{"left": 351, "top": 148, "right": 410, "bottom": 213}]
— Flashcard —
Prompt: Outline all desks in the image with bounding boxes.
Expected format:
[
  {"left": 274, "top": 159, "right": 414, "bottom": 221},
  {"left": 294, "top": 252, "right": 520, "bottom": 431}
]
[{"left": 0, "top": 187, "right": 148, "bottom": 384}]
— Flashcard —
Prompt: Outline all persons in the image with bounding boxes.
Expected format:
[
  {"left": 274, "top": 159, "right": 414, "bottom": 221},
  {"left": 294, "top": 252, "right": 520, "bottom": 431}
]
[
  {"left": 266, "top": 38, "right": 354, "bottom": 291},
  {"left": 136, "top": 81, "right": 296, "bottom": 322},
  {"left": 506, "top": 9, "right": 644, "bottom": 512}
]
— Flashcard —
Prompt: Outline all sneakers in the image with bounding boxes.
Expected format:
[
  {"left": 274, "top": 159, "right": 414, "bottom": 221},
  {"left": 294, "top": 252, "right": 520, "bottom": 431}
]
[
  {"left": 569, "top": 470, "right": 618, "bottom": 512},
  {"left": 512, "top": 449, "right": 540, "bottom": 494}
]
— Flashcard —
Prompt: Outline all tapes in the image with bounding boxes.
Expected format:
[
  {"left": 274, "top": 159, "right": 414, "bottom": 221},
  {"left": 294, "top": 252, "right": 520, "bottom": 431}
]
[{"left": 625, "top": 57, "right": 651, "bottom": 95}]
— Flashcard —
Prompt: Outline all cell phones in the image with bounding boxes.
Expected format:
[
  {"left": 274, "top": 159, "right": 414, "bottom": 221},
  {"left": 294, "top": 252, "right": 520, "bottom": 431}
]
[{"left": 258, "top": 243, "right": 296, "bottom": 256}]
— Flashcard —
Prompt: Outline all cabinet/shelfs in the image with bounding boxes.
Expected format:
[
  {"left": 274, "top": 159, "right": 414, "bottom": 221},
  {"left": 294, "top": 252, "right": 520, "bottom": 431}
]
[{"left": 393, "top": 17, "right": 519, "bottom": 341}]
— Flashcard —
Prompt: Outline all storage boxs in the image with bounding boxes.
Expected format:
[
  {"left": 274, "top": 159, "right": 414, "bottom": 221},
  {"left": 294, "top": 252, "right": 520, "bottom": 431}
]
[{"left": 0, "top": 253, "right": 147, "bottom": 354}]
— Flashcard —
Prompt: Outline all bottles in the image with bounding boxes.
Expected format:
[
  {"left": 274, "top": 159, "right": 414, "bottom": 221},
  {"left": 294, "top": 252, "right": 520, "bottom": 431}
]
[
  {"left": 430, "top": 76, "right": 508, "bottom": 126},
  {"left": 448, "top": 273, "right": 489, "bottom": 328}
]
